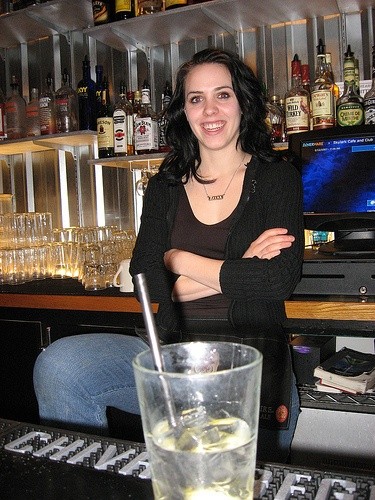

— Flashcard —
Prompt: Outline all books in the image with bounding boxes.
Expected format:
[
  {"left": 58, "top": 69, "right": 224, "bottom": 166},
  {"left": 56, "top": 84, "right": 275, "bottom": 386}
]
[{"left": 314, "top": 347, "right": 375, "bottom": 394}]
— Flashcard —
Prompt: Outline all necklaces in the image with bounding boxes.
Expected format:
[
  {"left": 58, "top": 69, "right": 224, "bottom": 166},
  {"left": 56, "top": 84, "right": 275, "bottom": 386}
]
[{"left": 199, "top": 154, "right": 245, "bottom": 201}]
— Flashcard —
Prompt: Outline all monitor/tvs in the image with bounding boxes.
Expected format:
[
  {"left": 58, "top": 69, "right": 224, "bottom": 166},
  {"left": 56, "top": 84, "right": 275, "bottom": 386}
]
[{"left": 289, "top": 124, "right": 375, "bottom": 260}]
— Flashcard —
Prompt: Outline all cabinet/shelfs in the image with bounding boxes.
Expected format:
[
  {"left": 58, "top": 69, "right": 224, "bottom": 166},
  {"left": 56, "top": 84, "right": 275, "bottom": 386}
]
[{"left": 0, "top": 0, "right": 375, "bottom": 445}]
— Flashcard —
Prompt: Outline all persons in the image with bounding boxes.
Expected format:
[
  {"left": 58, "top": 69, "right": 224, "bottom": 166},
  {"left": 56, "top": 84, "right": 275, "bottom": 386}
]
[{"left": 33, "top": 48, "right": 304, "bottom": 463}]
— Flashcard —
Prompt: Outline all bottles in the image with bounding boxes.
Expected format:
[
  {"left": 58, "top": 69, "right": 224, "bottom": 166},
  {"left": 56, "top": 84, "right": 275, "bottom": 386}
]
[
  {"left": 92, "top": 0, "right": 188, "bottom": 27},
  {"left": 266, "top": 37, "right": 375, "bottom": 141},
  {"left": 312, "top": 230, "right": 328, "bottom": 251},
  {"left": 132, "top": 342, "right": 263, "bottom": 500},
  {"left": 0, "top": 56, "right": 174, "bottom": 152}
]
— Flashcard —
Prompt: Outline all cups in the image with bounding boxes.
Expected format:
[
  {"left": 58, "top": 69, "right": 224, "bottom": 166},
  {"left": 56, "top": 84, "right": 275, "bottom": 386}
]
[
  {"left": 113, "top": 259, "right": 134, "bottom": 293},
  {"left": 0, "top": 193, "right": 136, "bottom": 291}
]
